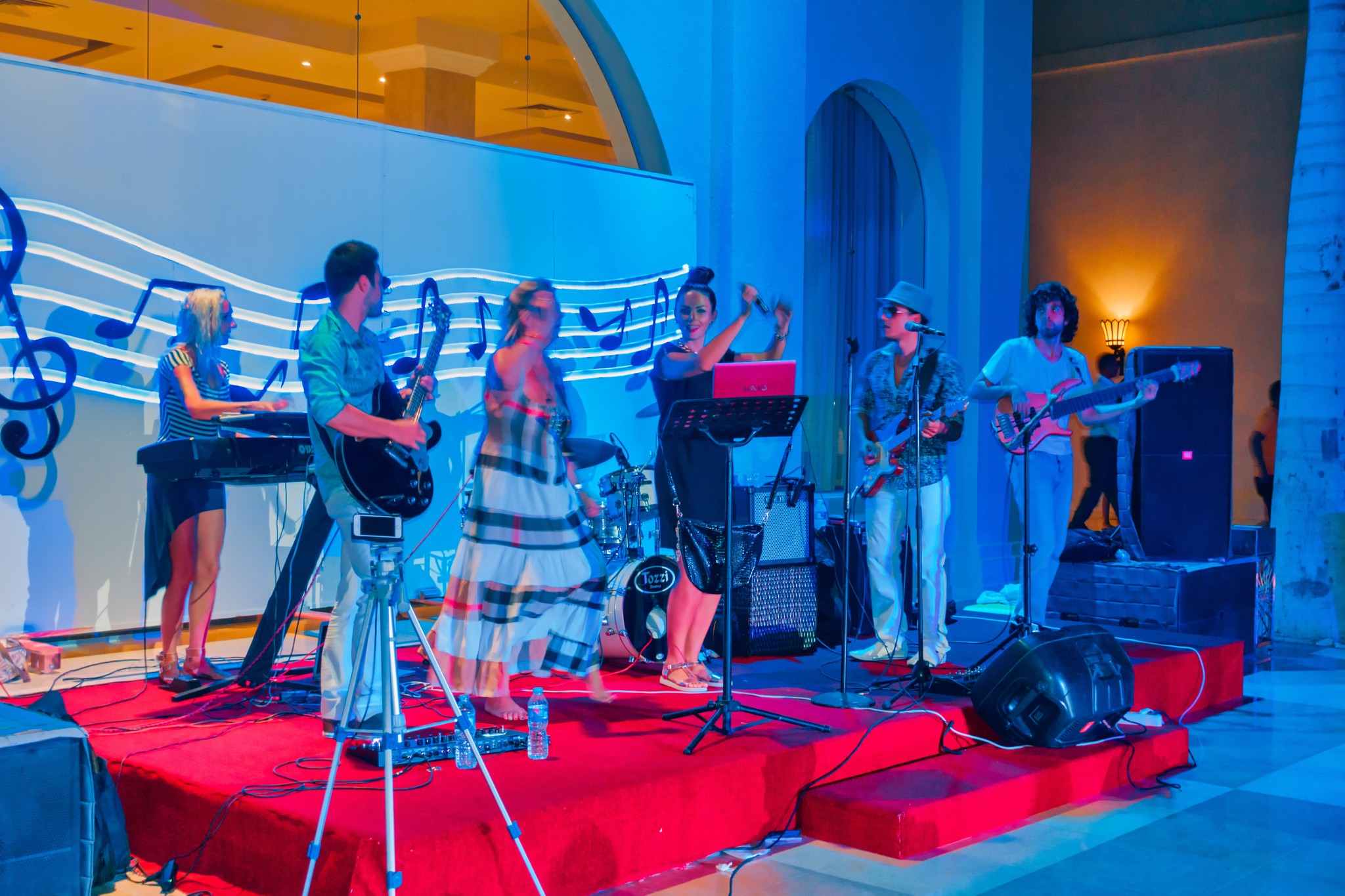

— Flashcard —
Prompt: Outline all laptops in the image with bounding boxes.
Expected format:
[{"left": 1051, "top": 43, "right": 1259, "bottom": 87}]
[{"left": 713, "top": 360, "right": 797, "bottom": 419}]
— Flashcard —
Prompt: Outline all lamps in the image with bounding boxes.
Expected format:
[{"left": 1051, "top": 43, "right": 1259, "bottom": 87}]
[{"left": 1098, "top": 319, "right": 1129, "bottom": 379}]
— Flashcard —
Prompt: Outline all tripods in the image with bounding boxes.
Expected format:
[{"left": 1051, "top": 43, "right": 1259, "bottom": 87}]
[
  {"left": 872, "top": 332, "right": 977, "bottom": 709},
  {"left": 657, "top": 395, "right": 833, "bottom": 755},
  {"left": 302, "top": 539, "right": 546, "bottom": 896}
]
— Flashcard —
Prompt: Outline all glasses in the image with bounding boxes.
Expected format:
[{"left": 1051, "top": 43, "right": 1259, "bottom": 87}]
[
  {"left": 377, "top": 275, "right": 392, "bottom": 290},
  {"left": 877, "top": 305, "right": 906, "bottom": 319}
]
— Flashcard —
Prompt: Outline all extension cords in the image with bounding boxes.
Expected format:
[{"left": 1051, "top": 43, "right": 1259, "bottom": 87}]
[{"left": 1117, "top": 711, "right": 1162, "bottom": 727}]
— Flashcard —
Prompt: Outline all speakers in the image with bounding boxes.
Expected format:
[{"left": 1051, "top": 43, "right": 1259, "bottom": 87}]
[
  {"left": 971, "top": 624, "right": 1135, "bottom": 748},
  {"left": 733, "top": 483, "right": 816, "bottom": 568},
  {"left": 1126, "top": 345, "right": 1233, "bottom": 560}
]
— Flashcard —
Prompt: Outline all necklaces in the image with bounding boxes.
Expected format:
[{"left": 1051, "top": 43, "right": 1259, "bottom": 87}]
[{"left": 677, "top": 337, "right": 698, "bottom": 354}]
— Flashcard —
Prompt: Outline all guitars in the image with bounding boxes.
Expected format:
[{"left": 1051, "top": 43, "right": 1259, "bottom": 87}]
[
  {"left": 990, "top": 359, "right": 1202, "bottom": 456},
  {"left": 336, "top": 296, "right": 453, "bottom": 522},
  {"left": 858, "top": 395, "right": 970, "bottom": 499}
]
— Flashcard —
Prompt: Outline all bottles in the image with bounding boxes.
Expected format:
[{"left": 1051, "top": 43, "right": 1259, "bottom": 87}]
[
  {"left": 527, "top": 686, "right": 549, "bottom": 760},
  {"left": 454, "top": 694, "right": 477, "bottom": 769}
]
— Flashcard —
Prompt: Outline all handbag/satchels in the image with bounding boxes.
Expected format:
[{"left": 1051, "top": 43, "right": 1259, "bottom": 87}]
[
  {"left": 1057, "top": 527, "right": 1125, "bottom": 563},
  {"left": 676, "top": 515, "right": 765, "bottom": 595}
]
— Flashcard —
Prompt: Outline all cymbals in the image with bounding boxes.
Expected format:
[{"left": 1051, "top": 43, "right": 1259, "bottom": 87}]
[{"left": 557, "top": 437, "right": 616, "bottom": 471}]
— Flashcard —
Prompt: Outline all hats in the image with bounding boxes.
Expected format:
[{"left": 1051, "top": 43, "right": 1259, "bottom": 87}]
[{"left": 875, "top": 282, "right": 935, "bottom": 325}]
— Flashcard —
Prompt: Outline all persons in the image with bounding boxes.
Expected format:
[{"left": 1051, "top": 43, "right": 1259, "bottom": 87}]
[
  {"left": 143, "top": 287, "right": 287, "bottom": 687},
  {"left": 1248, "top": 379, "right": 1281, "bottom": 527},
  {"left": 848, "top": 278, "right": 965, "bottom": 669},
  {"left": 1067, "top": 352, "right": 1121, "bottom": 531},
  {"left": 650, "top": 266, "right": 792, "bottom": 693},
  {"left": 970, "top": 281, "right": 1157, "bottom": 633},
  {"left": 418, "top": 278, "right": 609, "bottom": 721},
  {"left": 298, "top": 241, "right": 434, "bottom": 738}
]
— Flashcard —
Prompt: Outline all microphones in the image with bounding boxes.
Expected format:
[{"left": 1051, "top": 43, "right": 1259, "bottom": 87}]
[
  {"left": 610, "top": 435, "right": 630, "bottom": 469},
  {"left": 905, "top": 321, "right": 945, "bottom": 336},
  {"left": 739, "top": 282, "right": 772, "bottom": 318},
  {"left": 790, "top": 467, "right": 806, "bottom": 506}
]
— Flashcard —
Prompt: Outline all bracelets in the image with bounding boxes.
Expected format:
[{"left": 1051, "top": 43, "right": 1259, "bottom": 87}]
[
  {"left": 775, "top": 324, "right": 789, "bottom": 342},
  {"left": 517, "top": 331, "right": 548, "bottom": 350}
]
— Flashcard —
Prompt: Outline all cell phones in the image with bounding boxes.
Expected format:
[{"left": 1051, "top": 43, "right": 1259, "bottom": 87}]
[{"left": 353, "top": 513, "right": 403, "bottom": 539}]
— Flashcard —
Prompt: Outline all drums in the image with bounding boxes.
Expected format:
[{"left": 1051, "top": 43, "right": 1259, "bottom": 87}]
[
  {"left": 597, "top": 553, "right": 681, "bottom": 664},
  {"left": 624, "top": 525, "right": 644, "bottom": 544},
  {"left": 600, "top": 464, "right": 660, "bottom": 526},
  {"left": 585, "top": 501, "right": 623, "bottom": 552},
  {"left": 601, "top": 544, "right": 629, "bottom": 560}
]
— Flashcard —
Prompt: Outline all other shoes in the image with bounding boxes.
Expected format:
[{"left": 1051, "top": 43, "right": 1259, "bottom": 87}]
[
  {"left": 907, "top": 645, "right": 947, "bottom": 667},
  {"left": 849, "top": 641, "right": 907, "bottom": 661},
  {"left": 322, "top": 713, "right": 383, "bottom": 738}
]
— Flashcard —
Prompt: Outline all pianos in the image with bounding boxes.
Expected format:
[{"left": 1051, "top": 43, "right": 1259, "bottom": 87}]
[{"left": 135, "top": 411, "right": 336, "bottom": 702}]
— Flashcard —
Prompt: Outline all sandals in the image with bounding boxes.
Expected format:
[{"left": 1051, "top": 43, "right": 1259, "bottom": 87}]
[
  {"left": 658, "top": 662, "right": 708, "bottom": 692},
  {"left": 183, "top": 647, "right": 232, "bottom": 682},
  {"left": 687, "top": 660, "right": 733, "bottom": 688},
  {"left": 156, "top": 651, "right": 200, "bottom": 687}
]
